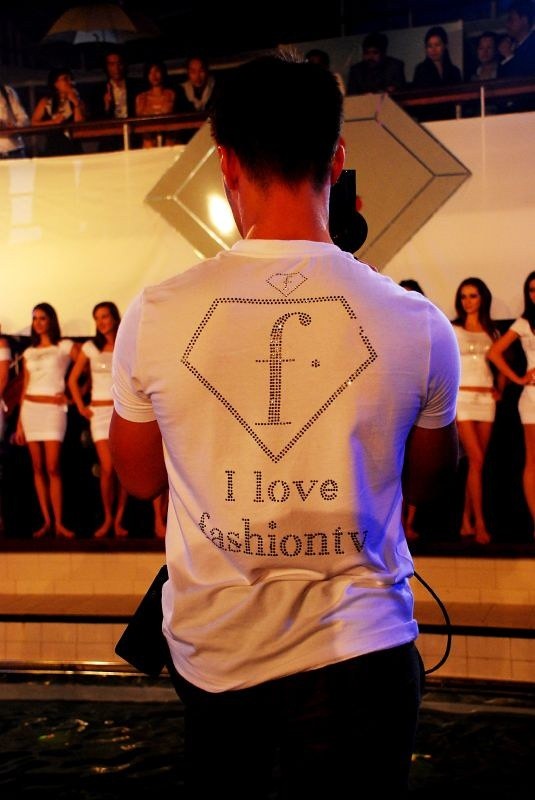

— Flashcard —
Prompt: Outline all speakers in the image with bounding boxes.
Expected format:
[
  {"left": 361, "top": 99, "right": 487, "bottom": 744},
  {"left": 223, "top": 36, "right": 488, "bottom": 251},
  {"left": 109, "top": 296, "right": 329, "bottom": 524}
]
[{"left": 114, "top": 568, "right": 169, "bottom": 677}]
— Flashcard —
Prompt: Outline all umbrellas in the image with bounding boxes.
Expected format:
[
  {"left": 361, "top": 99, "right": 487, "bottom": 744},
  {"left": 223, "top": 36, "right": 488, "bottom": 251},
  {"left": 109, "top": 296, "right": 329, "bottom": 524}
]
[{"left": 43, "top": 0, "right": 138, "bottom": 74}]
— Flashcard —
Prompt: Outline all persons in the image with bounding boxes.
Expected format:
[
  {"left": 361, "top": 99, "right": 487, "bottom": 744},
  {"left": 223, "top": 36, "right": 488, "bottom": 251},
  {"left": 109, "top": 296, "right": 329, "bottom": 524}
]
[
  {"left": 109, "top": 49, "right": 463, "bottom": 800},
  {"left": 0, "top": 270, "right": 535, "bottom": 546},
  {"left": 0, "top": 0, "right": 535, "bottom": 162}
]
